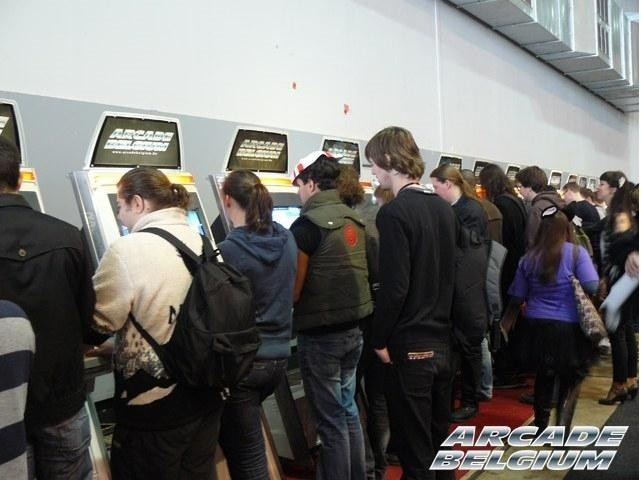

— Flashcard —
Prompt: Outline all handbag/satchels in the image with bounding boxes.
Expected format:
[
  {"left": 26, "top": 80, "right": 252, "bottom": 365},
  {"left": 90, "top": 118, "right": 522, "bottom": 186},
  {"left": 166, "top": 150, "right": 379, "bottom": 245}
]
[{"left": 571, "top": 244, "right": 612, "bottom": 356}]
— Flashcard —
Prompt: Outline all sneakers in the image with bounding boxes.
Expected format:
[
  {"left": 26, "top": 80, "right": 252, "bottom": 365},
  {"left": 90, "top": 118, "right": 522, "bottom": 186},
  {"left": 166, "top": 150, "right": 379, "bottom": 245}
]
[{"left": 450, "top": 372, "right": 528, "bottom": 422}]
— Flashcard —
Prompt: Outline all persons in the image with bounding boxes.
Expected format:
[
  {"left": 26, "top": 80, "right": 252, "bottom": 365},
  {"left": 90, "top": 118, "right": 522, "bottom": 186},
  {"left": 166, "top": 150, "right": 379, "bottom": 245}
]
[
  {"left": 0, "top": 300, "right": 36, "bottom": 480},
  {"left": 335, "top": 128, "right": 639, "bottom": 480},
  {"left": 0, "top": 133, "right": 95, "bottom": 480},
  {"left": 290, "top": 150, "right": 374, "bottom": 480},
  {"left": 92, "top": 169, "right": 225, "bottom": 480},
  {"left": 215, "top": 171, "right": 297, "bottom": 480}
]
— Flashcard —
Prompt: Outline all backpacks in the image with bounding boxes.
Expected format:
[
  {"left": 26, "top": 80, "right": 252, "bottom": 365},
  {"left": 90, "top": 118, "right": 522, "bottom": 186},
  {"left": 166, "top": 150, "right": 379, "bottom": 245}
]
[
  {"left": 128, "top": 227, "right": 261, "bottom": 402},
  {"left": 451, "top": 214, "right": 509, "bottom": 353}
]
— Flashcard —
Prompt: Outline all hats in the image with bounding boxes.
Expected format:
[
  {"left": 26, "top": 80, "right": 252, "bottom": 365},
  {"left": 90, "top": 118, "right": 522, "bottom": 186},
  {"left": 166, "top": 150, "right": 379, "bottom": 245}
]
[{"left": 289, "top": 151, "right": 344, "bottom": 186}]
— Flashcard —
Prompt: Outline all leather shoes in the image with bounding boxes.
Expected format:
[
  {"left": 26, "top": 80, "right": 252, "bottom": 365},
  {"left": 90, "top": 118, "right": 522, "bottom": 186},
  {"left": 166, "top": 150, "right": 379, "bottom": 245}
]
[{"left": 519, "top": 392, "right": 535, "bottom": 404}]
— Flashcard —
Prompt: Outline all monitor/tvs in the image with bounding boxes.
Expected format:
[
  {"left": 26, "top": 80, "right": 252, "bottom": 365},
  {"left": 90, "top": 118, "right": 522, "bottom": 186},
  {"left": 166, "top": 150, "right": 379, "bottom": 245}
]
[
  {"left": 270, "top": 192, "right": 303, "bottom": 229},
  {"left": 107, "top": 193, "right": 213, "bottom": 240}
]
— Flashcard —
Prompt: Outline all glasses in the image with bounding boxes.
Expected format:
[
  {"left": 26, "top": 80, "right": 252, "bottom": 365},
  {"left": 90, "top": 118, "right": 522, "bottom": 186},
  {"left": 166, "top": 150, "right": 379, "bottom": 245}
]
[{"left": 517, "top": 185, "right": 522, "bottom": 191}]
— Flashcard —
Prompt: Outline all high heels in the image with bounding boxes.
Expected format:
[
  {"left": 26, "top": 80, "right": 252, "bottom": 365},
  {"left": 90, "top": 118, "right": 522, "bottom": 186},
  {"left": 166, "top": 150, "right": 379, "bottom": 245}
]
[
  {"left": 599, "top": 391, "right": 627, "bottom": 406},
  {"left": 627, "top": 383, "right": 638, "bottom": 400}
]
